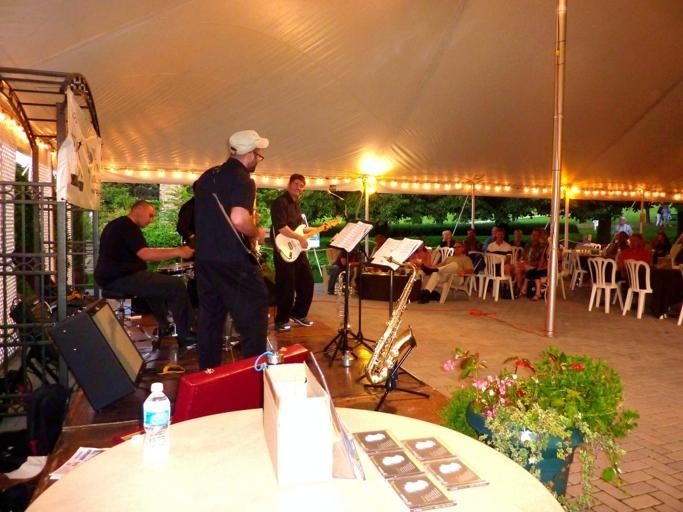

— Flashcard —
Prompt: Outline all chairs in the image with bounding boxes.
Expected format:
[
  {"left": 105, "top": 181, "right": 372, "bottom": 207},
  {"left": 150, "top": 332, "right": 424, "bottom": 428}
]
[
  {"left": 622, "top": 258, "right": 652, "bottom": 319},
  {"left": 438, "top": 247, "right": 453, "bottom": 261},
  {"left": 422, "top": 250, "right": 484, "bottom": 305},
  {"left": 540, "top": 245, "right": 567, "bottom": 304},
  {"left": 321, "top": 248, "right": 346, "bottom": 295},
  {"left": 484, "top": 253, "right": 515, "bottom": 301},
  {"left": 588, "top": 258, "right": 624, "bottom": 315},
  {"left": 569, "top": 253, "right": 589, "bottom": 290}
]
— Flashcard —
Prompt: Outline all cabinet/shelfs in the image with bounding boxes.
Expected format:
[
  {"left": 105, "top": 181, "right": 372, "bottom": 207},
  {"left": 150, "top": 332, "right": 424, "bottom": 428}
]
[{"left": 0, "top": 68, "right": 101, "bottom": 451}]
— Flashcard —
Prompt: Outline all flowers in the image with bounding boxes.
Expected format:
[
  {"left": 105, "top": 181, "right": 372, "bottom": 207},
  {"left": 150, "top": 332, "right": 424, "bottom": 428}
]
[{"left": 434, "top": 348, "right": 639, "bottom": 510}]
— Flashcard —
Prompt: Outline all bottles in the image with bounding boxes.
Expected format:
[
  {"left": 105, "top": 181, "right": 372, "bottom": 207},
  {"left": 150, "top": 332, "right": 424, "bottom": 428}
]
[
  {"left": 266, "top": 350, "right": 284, "bottom": 366},
  {"left": 143, "top": 382, "right": 171, "bottom": 436},
  {"left": 173, "top": 262, "right": 183, "bottom": 277}
]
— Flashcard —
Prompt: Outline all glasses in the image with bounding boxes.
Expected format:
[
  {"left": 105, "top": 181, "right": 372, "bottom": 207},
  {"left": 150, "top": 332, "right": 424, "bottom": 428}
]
[
  {"left": 453, "top": 245, "right": 463, "bottom": 249},
  {"left": 254, "top": 151, "right": 264, "bottom": 161}
]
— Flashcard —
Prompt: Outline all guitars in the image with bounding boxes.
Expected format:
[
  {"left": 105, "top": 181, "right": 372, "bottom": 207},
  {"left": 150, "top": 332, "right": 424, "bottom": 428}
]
[{"left": 274, "top": 215, "right": 344, "bottom": 263}]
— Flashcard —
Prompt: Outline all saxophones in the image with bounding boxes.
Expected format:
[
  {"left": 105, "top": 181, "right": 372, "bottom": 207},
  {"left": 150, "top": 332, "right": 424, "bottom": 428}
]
[{"left": 365, "top": 252, "right": 421, "bottom": 391}]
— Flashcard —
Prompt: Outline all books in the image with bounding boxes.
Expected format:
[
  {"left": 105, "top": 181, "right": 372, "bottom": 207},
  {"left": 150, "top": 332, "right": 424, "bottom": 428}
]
[
  {"left": 329, "top": 221, "right": 373, "bottom": 252},
  {"left": 49, "top": 446, "right": 108, "bottom": 480},
  {"left": 370, "top": 237, "right": 424, "bottom": 271}
]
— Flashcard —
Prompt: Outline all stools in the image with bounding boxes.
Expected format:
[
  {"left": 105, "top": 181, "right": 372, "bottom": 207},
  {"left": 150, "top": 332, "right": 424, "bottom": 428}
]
[{"left": 103, "top": 290, "right": 159, "bottom": 350}]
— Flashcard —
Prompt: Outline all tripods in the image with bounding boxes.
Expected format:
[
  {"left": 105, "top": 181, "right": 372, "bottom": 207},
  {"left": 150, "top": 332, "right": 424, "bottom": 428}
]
[
  {"left": 323, "top": 249, "right": 374, "bottom": 367},
  {"left": 356, "top": 267, "right": 431, "bottom": 412}
]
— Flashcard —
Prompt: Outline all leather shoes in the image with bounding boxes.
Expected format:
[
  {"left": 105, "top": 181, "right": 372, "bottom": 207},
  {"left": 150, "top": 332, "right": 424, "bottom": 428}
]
[
  {"left": 421, "top": 290, "right": 431, "bottom": 299},
  {"left": 420, "top": 264, "right": 432, "bottom": 275}
]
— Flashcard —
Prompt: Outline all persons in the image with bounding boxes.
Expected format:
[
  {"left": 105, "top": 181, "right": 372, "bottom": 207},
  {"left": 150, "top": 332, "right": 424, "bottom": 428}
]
[
  {"left": 505, "top": 230, "right": 541, "bottom": 298},
  {"left": 519, "top": 228, "right": 551, "bottom": 301},
  {"left": 194, "top": 130, "right": 268, "bottom": 370},
  {"left": 409, "top": 242, "right": 430, "bottom": 268},
  {"left": 440, "top": 231, "right": 456, "bottom": 248},
  {"left": 325, "top": 242, "right": 360, "bottom": 295},
  {"left": 656, "top": 206, "right": 664, "bottom": 227},
  {"left": 271, "top": 174, "right": 328, "bottom": 332},
  {"left": 593, "top": 219, "right": 599, "bottom": 230},
  {"left": 615, "top": 217, "right": 633, "bottom": 235},
  {"left": 483, "top": 227, "right": 497, "bottom": 250},
  {"left": 616, "top": 233, "right": 652, "bottom": 311},
  {"left": 487, "top": 229, "right": 513, "bottom": 292},
  {"left": 575, "top": 235, "right": 591, "bottom": 250},
  {"left": 370, "top": 233, "right": 386, "bottom": 258},
  {"left": 509, "top": 229, "right": 528, "bottom": 254},
  {"left": 604, "top": 231, "right": 629, "bottom": 259},
  {"left": 650, "top": 227, "right": 672, "bottom": 256},
  {"left": 663, "top": 205, "right": 669, "bottom": 223},
  {"left": 463, "top": 229, "right": 484, "bottom": 289},
  {"left": 177, "top": 181, "right": 198, "bottom": 248},
  {"left": 418, "top": 240, "right": 474, "bottom": 304},
  {"left": 94, "top": 201, "right": 198, "bottom": 349}
]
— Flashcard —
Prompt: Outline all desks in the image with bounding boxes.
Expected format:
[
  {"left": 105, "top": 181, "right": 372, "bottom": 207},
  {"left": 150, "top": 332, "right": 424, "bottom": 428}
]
[
  {"left": 358, "top": 273, "right": 422, "bottom": 302},
  {"left": 641, "top": 266, "right": 682, "bottom": 319},
  {"left": 26, "top": 407, "right": 563, "bottom": 511}
]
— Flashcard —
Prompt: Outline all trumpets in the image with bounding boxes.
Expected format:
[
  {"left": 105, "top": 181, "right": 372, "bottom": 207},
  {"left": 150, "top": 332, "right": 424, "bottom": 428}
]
[{"left": 334, "top": 270, "right": 353, "bottom": 330}]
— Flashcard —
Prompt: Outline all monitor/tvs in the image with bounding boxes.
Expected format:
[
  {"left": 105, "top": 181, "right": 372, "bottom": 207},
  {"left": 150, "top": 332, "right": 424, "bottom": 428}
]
[{"left": 51, "top": 300, "right": 144, "bottom": 414}]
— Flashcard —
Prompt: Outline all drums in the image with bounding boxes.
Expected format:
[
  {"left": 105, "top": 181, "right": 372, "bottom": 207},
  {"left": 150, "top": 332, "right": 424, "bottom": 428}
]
[{"left": 157, "top": 262, "right": 196, "bottom": 284}]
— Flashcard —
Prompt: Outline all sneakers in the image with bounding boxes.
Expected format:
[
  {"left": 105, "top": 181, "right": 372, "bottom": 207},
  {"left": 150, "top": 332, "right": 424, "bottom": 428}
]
[
  {"left": 276, "top": 321, "right": 291, "bottom": 331},
  {"left": 291, "top": 312, "right": 313, "bottom": 327}
]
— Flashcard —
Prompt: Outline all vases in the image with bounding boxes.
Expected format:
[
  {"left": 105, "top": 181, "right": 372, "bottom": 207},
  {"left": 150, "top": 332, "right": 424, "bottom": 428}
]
[{"left": 465, "top": 404, "right": 584, "bottom": 499}]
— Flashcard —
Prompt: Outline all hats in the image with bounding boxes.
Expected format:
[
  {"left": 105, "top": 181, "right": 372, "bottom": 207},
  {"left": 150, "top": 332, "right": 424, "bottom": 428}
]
[{"left": 229, "top": 130, "right": 269, "bottom": 155}]
